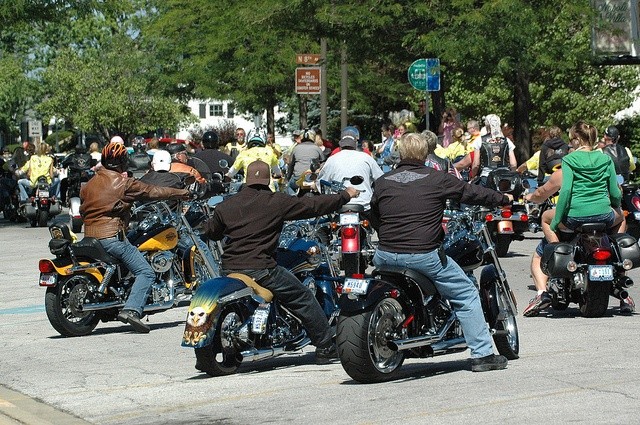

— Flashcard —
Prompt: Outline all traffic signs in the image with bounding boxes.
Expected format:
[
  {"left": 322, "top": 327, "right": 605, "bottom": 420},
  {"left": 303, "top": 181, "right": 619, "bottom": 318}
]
[{"left": 296, "top": 53, "right": 320, "bottom": 65}]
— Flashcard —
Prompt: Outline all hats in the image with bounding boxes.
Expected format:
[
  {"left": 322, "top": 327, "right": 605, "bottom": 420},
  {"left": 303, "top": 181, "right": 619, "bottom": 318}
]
[
  {"left": 341, "top": 131, "right": 356, "bottom": 147},
  {"left": 293, "top": 130, "right": 300, "bottom": 136},
  {"left": 151, "top": 150, "right": 172, "bottom": 172},
  {"left": 246, "top": 161, "right": 270, "bottom": 186},
  {"left": 165, "top": 143, "right": 187, "bottom": 154}
]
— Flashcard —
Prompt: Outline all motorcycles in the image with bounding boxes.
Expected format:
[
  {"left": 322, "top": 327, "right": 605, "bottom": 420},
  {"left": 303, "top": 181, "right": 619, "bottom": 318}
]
[
  {"left": 328, "top": 203, "right": 375, "bottom": 276},
  {"left": 522, "top": 173, "right": 640, "bottom": 317},
  {"left": 67, "top": 179, "right": 83, "bottom": 232},
  {"left": 181, "top": 172, "right": 366, "bottom": 376},
  {"left": 39, "top": 175, "right": 220, "bottom": 336},
  {"left": 20, "top": 174, "right": 62, "bottom": 225},
  {"left": 473, "top": 166, "right": 530, "bottom": 256},
  {"left": 336, "top": 200, "right": 519, "bottom": 383}
]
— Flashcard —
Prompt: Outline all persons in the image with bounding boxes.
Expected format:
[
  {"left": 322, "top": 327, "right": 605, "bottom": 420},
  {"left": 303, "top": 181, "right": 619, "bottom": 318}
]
[
  {"left": 448, "top": 151, "right": 474, "bottom": 177},
  {"left": 522, "top": 125, "right": 569, "bottom": 217},
  {"left": 0, "top": 138, "right": 93, "bottom": 215},
  {"left": 446, "top": 127, "right": 475, "bottom": 158},
  {"left": 361, "top": 140, "right": 374, "bottom": 152},
  {"left": 110, "top": 136, "right": 125, "bottom": 146},
  {"left": 191, "top": 131, "right": 243, "bottom": 174},
  {"left": 517, "top": 148, "right": 540, "bottom": 177},
  {"left": 314, "top": 134, "right": 330, "bottom": 159},
  {"left": 438, "top": 106, "right": 464, "bottom": 148},
  {"left": 393, "top": 124, "right": 407, "bottom": 139},
  {"left": 202, "top": 159, "right": 360, "bottom": 365},
  {"left": 566, "top": 141, "right": 573, "bottom": 152},
  {"left": 146, "top": 138, "right": 159, "bottom": 154},
  {"left": 78, "top": 143, "right": 190, "bottom": 334},
  {"left": 330, "top": 126, "right": 371, "bottom": 157},
  {"left": 88, "top": 142, "right": 102, "bottom": 160},
  {"left": 418, "top": 99, "right": 438, "bottom": 133},
  {"left": 460, "top": 114, "right": 517, "bottom": 211},
  {"left": 224, "top": 128, "right": 248, "bottom": 155},
  {"left": 435, "top": 144, "right": 447, "bottom": 158},
  {"left": 313, "top": 130, "right": 384, "bottom": 220},
  {"left": 595, "top": 125, "right": 636, "bottom": 185},
  {"left": 137, "top": 149, "right": 224, "bottom": 216},
  {"left": 418, "top": 130, "right": 449, "bottom": 172},
  {"left": 367, "top": 130, "right": 515, "bottom": 373},
  {"left": 128, "top": 136, "right": 151, "bottom": 173},
  {"left": 284, "top": 128, "right": 326, "bottom": 195},
  {"left": 315, "top": 128, "right": 333, "bottom": 147},
  {"left": 522, "top": 141, "right": 637, "bottom": 315},
  {"left": 376, "top": 123, "right": 393, "bottom": 172},
  {"left": 266, "top": 132, "right": 282, "bottom": 160},
  {"left": 224, "top": 126, "right": 281, "bottom": 192},
  {"left": 165, "top": 143, "right": 208, "bottom": 183},
  {"left": 466, "top": 121, "right": 482, "bottom": 150},
  {"left": 284, "top": 130, "right": 303, "bottom": 162},
  {"left": 540, "top": 119, "right": 627, "bottom": 243}
]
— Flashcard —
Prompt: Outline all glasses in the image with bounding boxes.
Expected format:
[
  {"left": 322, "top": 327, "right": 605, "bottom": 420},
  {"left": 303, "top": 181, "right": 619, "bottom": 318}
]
[{"left": 236, "top": 133, "right": 244, "bottom": 136}]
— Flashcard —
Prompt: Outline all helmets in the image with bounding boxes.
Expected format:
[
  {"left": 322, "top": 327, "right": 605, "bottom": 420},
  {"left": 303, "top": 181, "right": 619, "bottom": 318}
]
[
  {"left": 202, "top": 131, "right": 218, "bottom": 149},
  {"left": 300, "top": 126, "right": 316, "bottom": 143},
  {"left": 132, "top": 135, "right": 148, "bottom": 152},
  {"left": 343, "top": 127, "right": 360, "bottom": 141},
  {"left": 101, "top": 142, "right": 128, "bottom": 169},
  {"left": 75, "top": 144, "right": 84, "bottom": 154},
  {"left": 111, "top": 136, "right": 124, "bottom": 145},
  {"left": 247, "top": 126, "right": 268, "bottom": 149}
]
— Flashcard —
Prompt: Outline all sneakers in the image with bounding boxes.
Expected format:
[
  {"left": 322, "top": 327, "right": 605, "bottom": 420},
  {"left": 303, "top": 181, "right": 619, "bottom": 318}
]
[
  {"left": 313, "top": 343, "right": 342, "bottom": 365},
  {"left": 522, "top": 294, "right": 552, "bottom": 316},
  {"left": 620, "top": 295, "right": 635, "bottom": 315},
  {"left": 471, "top": 353, "right": 508, "bottom": 371},
  {"left": 117, "top": 309, "right": 150, "bottom": 333}
]
